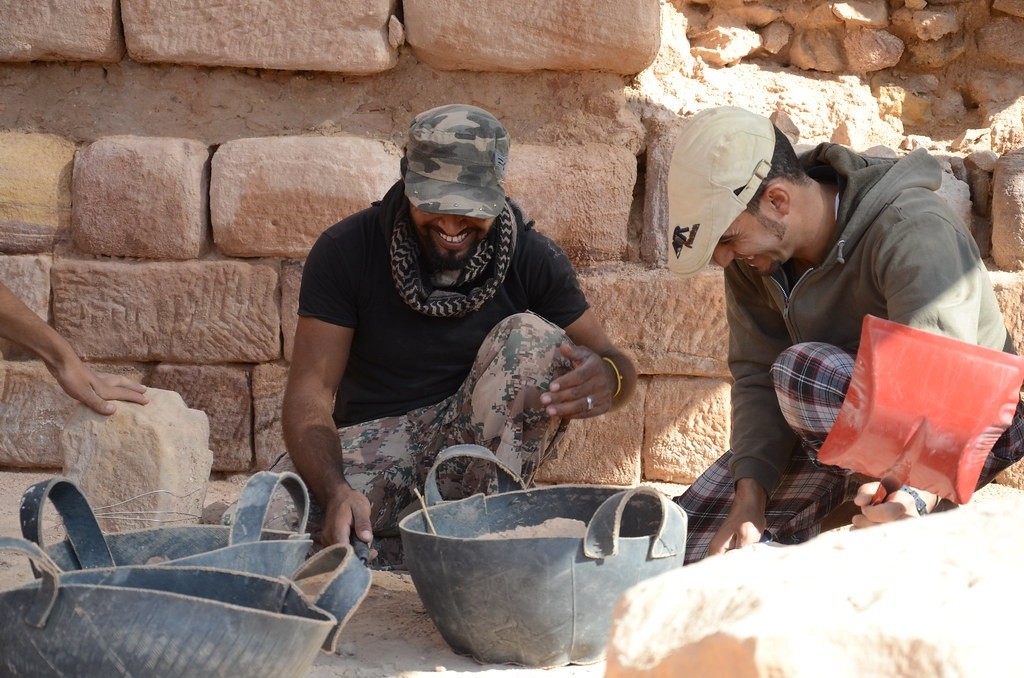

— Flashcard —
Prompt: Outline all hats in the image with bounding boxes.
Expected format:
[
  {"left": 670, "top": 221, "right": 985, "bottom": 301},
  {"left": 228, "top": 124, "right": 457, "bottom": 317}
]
[
  {"left": 667, "top": 105, "right": 774, "bottom": 279},
  {"left": 403, "top": 104, "right": 509, "bottom": 220}
]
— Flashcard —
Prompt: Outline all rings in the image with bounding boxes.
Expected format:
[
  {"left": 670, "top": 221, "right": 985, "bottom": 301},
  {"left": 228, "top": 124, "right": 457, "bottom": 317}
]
[{"left": 586, "top": 395, "right": 594, "bottom": 410}]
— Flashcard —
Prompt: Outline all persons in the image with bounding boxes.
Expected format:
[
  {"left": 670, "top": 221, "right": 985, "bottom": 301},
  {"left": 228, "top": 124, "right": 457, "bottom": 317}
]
[
  {"left": 198, "top": 104, "right": 637, "bottom": 572},
  {"left": 0, "top": 281, "right": 149, "bottom": 416},
  {"left": 669, "top": 107, "right": 1024, "bottom": 567}
]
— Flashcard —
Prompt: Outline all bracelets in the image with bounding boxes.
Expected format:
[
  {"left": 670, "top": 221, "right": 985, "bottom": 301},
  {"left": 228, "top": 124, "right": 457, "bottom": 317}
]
[
  {"left": 602, "top": 357, "right": 622, "bottom": 399},
  {"left": 898, "top": 485, "right": 927, "bottom": 516}
]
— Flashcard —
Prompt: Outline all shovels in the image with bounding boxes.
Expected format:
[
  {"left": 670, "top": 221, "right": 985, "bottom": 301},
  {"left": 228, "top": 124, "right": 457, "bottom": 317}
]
[{"left": 816, "top": 314, "right": 1024, "bottom": 507}]
